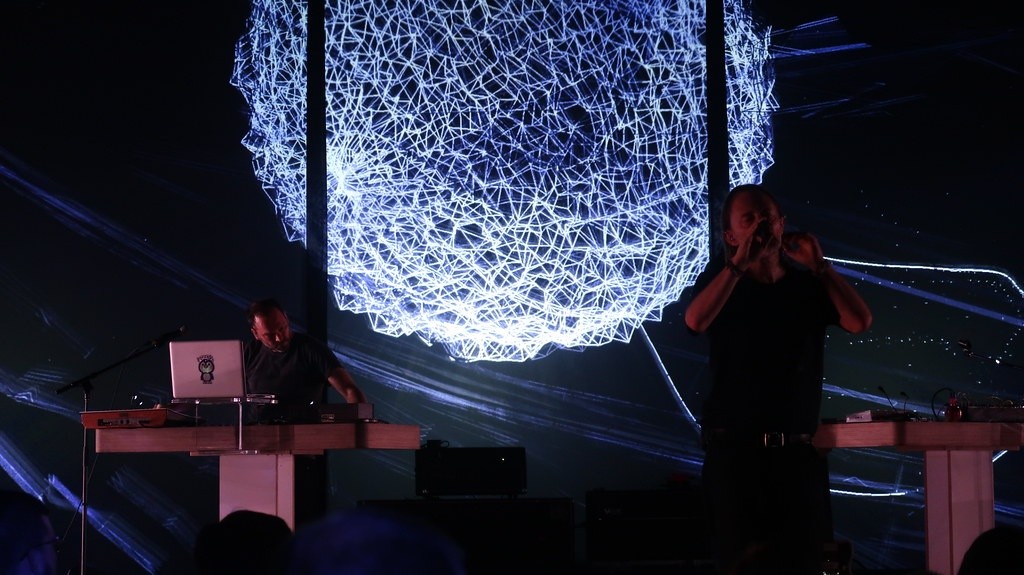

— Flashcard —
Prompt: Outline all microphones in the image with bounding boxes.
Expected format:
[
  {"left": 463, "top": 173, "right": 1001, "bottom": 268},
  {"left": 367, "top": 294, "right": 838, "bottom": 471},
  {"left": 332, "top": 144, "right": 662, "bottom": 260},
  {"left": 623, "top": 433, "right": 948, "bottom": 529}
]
[
  {"left": 754, "top": 220, "right": 770, "bottom": 239},
  {"left": 900, "top": 392, "right": 909, "bottom": 413},
  {"left": 145, "top": 325, "right": 187, "bottom": 347},
  {"left": 878, "top": 385, "right": 897, "bottom": 413}
]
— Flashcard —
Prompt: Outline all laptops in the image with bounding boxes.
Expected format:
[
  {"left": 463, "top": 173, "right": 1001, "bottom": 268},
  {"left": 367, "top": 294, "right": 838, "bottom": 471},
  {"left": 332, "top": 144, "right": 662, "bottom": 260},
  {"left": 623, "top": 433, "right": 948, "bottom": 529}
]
[{"left": 169, "top": 339, "right": 275, "bottom": 398}]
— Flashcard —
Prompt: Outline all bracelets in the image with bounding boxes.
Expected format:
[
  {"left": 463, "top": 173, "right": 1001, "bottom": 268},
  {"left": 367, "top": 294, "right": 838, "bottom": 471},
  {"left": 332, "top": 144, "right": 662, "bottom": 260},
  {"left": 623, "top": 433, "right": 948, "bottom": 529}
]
[{"left": 725, "top": 260, "right": 744, "bottom": 277}]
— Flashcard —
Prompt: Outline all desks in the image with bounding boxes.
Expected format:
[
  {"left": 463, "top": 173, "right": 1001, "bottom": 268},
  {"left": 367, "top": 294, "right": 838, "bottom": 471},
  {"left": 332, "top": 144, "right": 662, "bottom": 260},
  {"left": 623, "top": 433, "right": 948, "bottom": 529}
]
[
  {"left": 96, "top": 424, "right": 419, "bottom": 533},
  {"left": 812, "top": 423, "right": 1024, "bottom": 575}
]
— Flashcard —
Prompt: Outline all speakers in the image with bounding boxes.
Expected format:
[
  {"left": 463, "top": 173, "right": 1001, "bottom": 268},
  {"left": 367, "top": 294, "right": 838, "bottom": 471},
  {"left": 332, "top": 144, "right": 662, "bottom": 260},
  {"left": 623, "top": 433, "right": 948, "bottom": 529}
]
[{"left": 414, "top": 447, "right": 526, "bottom": 497}]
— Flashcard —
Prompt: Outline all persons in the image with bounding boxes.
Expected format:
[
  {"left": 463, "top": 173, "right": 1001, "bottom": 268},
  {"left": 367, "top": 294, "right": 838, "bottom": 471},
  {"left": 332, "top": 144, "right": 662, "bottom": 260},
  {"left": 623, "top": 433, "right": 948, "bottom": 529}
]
[
  {"left": 683, "top": 185, "right": 873, "bottom": 575},
  {"left": 194, "top": 511, "right": 291, "bottom": 575},
  {"left": 729, "top": 540, "right": 853, "bottom": 575},
  {"left": 292, "top": 512, "right": 465, "bottom": 575},
  {"left": 0, "top": 489, "right": 59, "bottom": 575},
  {"left": 958, "top": 527, "right": 1024, "bottom": 575},
  {"left": 155, "top": 299, "right": 378, "bottom": 527}
]
